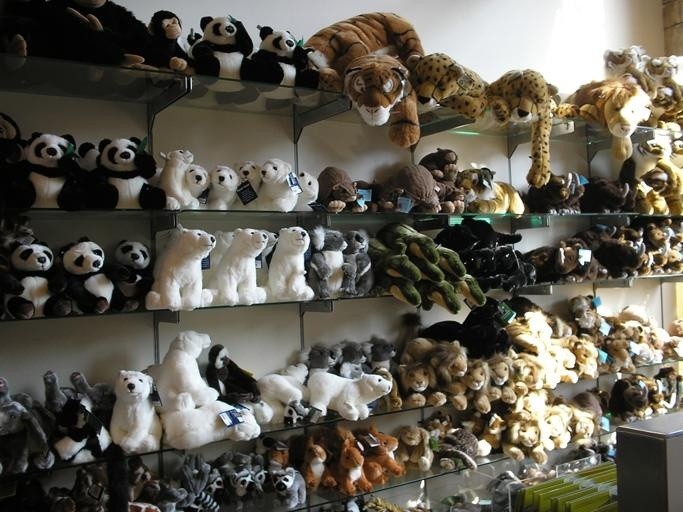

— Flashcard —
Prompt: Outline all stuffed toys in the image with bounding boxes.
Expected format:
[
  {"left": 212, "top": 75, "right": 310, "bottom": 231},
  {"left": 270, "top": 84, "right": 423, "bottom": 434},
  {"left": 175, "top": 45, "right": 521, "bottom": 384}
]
[{"left": 0, "top": 1, "right": 683, "bottom": 512}]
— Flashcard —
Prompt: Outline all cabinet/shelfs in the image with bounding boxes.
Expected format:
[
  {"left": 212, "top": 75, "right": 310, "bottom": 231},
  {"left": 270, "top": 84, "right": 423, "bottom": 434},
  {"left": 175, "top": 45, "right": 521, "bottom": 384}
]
[{"left": 1, "top": 50, "right": 682, "bottom": 510}]
